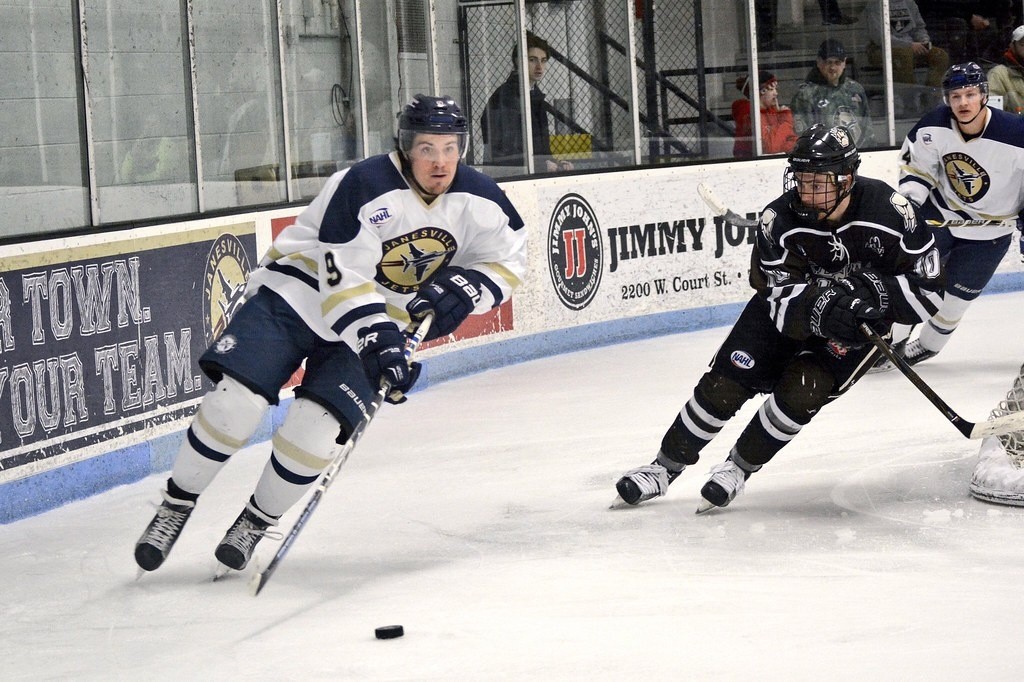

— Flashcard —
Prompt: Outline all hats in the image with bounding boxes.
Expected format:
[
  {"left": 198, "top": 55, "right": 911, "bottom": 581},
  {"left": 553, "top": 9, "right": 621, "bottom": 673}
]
[
  {"left": 736, "top": 71, "right": 778, "bottom": 99},
  {"left": 818, "top": 39, "right": 845, "bottom": 61},
  {"left": 512, "top": 29, "right": 550, "bottom": 64},
  {"left": 1012, "top": 25, "right": 1024, "bottom": 42}
]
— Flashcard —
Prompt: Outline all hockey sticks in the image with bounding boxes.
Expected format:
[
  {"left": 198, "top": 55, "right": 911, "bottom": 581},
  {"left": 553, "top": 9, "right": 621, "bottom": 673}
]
[
  {"left": 856, "top": 317, "right": 1024, "bottom": 440},
  {"left": 244, "top": 315, "right": 434, "bottom": 596},
  {"left": 695, "top": 178, "right": 1015, "bottom": 230}
]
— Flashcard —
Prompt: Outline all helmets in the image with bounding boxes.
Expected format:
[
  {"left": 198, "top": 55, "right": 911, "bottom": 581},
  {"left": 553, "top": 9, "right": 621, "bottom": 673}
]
[
  {"left": 941, "top": 62, "right": 989, "bottom": 106},
  {"left": 397, "top": 94, "right": 469, "bottom": 162},
  {"left": 782, "top": 123, "right": 861, "bottom": 222}
]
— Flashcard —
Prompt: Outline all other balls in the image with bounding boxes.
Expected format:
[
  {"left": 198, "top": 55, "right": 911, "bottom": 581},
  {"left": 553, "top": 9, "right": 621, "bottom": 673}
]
[{"left": 374, "top": 624, "right": 404, "bottom": 640}]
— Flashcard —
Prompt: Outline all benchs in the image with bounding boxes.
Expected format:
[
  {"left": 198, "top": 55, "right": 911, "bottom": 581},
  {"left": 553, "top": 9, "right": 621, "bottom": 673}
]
[{"left": 709, "top": 0, "right": 935, "bottom": 125}]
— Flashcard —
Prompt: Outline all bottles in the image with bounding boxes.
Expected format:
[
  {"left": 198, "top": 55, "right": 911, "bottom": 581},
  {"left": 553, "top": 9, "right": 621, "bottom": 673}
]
[{"left": 1015, "top": 107, "right": 1022, "bottom": 115}]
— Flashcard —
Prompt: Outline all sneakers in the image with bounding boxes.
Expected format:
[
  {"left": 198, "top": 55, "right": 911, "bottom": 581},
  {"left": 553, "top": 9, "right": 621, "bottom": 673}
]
[
  {"left": 133, "top": 477, "right": 200, "bottom": 581},
  {"left": 608, "top": 458, "right": 682, "bottom": 510},
  {"left": 901, "top": 338, "right": 939, "bottom": 367},
  {"left": 866, "top": 337, "right": 910, "bottom": 374},
  {"left": 695, "top": 456, "right": 751, "bottom": 514},
  {"left": 213, "top": 493, "right": 284, "bottom": 582}
]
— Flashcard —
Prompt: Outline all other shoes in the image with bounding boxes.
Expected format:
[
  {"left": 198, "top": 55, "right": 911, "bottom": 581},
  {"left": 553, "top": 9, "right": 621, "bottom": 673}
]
[
  {"left": 822, "top": 14, "right": 859, "bottom": 25},
  {"left": 757, "top": 39, "right": 792, "bottom": 50}
]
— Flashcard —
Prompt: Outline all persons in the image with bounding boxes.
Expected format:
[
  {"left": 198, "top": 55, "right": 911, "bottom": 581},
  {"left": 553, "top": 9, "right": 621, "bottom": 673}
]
[
  {"left": 732, "top": 70, "right": 799, "bottom": 160},
  {"left": 616, "top": 125, "right": 945, "bottom": 507},
  {"left": 486, "top": 36, "right": 552, "bottom": 166},
  {"left": 817, "top": 0, "right": 859, "bottom": 25},
  {"left": 133, "top": 95, "right": 529, "bottom": 572},
  {"left": 120, "top": 111, "right": 181, "bottom": 184},
  {"left": 870, "top": 62, "right": 1024, "bottom": 370},
  {"left": 968, "top": 364, "right": 1024, "bottom": 507},
  {"left": 944, "top": 0, "right": 1024, "bottom": 116},
  {"left": 790, "top": 38, "right": 878, "bottom": 149},
  {"left": 865, "top": 0, "right": 949, "bottom": 119},
  {"left": 218, "top": 72, "right": 267, "bottom": 177},
  {"left": 756, "top": 0, "right": 793, "bottom": 52}
]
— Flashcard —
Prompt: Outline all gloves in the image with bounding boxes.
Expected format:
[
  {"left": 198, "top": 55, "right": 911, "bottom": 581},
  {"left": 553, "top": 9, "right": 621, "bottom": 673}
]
[
  {"left": 406, "top": 266, "right": 482, "bottom": 342},
  {"left": 806, "top": 269, "right": 889, "bottom": 340},
  {"left": 357, "top": 322, "right": 422, "bottom": 404}
]
